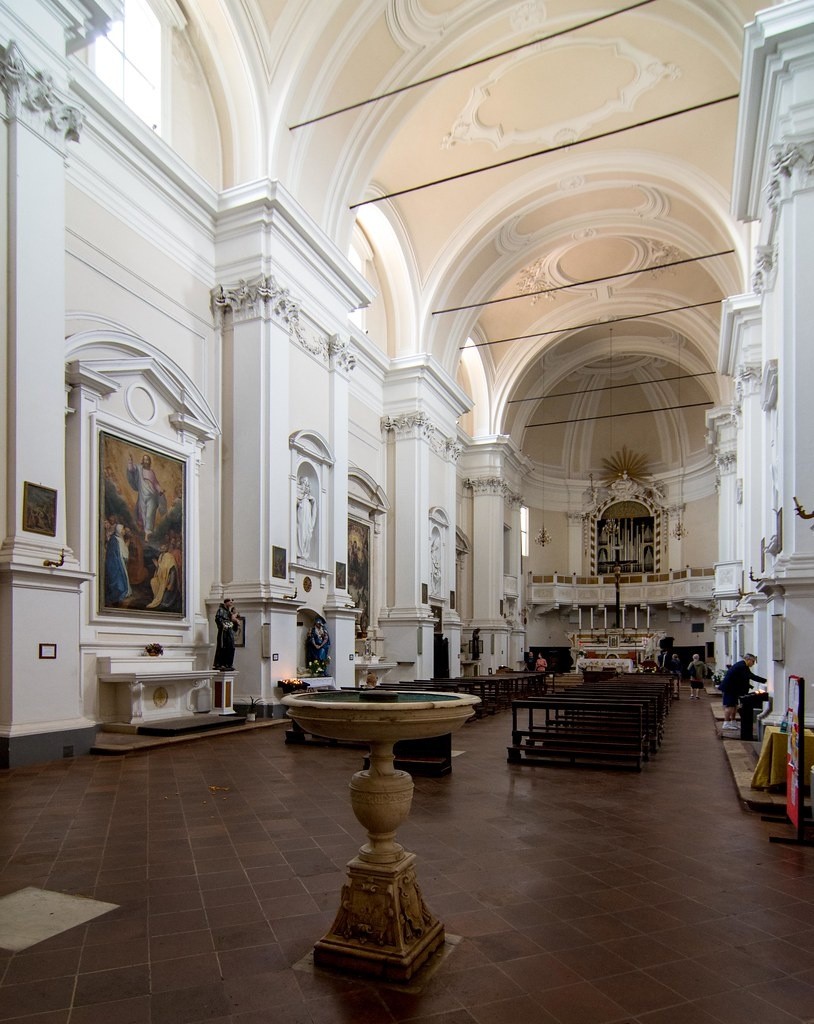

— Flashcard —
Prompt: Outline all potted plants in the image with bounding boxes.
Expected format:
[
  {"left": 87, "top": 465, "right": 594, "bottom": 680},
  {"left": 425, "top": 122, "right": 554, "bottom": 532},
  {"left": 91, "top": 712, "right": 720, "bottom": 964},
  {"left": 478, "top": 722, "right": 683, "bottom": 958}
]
[
  {"left": 240, "top": 695, "right": 264, "bottom": 721},
  {"left": 146, "top": 643, "right": 163, "bottom": 656}
]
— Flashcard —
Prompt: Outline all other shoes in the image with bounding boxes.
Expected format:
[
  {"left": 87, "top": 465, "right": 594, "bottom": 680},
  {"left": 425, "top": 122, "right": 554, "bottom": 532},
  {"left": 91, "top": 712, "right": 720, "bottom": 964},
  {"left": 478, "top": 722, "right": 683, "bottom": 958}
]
[
  {"left": 734, "top": 725, "right": 741, "bottom": 728},
  {"left": 690, "top": 696, "right": 695, "bottom": 699},
  {"left": 722, "top": 726, "right": 738, "bottom": 730},
  {"left": 696, "top": 697, "right": 700, "bottom": 700}
]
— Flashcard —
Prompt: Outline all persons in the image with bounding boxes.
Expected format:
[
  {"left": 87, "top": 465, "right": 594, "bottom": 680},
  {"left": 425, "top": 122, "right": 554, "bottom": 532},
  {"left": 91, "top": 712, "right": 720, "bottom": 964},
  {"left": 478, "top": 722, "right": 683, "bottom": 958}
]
[
  {"left": 306, "top": 620, "right": 332, "bottom": 676},
  {"left": 212, "top": 598, "right": 240, "bottom": 670},
  {"left": 670, "top": 654, "right": 684, "bottom": 694},
  {"left": 297, "top": 475, "right": 317, "bottom": 559},
  {"left": 657, "top": 649, "right": 672, "bottom": 671},
  {"left": 470, "top": 628, "right": 481, "bottom": 659},
  {"left": 535, "top": 653, "right": 547, "bottom": 671},
  {"left": 686, "top": 654, "right": 707, "bottom": 700},
  {"left": 717, "top": 654, "right": 767, "bottom": 730},
  {"left": 524, "top": 651, "right": 536, "bottom": 671}
]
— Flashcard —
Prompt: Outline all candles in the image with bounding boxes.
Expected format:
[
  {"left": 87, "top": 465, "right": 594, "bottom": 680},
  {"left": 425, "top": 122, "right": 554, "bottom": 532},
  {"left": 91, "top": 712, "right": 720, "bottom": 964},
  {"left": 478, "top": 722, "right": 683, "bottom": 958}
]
[
  {"left": 62, "top": 549, "right": 65, "bottom": 554},
  {"left": 793, "top": 497, "right": 799, "bottom": 507},
  {"left": 578, "top": 607, "right": 650, "bottom": 628}
]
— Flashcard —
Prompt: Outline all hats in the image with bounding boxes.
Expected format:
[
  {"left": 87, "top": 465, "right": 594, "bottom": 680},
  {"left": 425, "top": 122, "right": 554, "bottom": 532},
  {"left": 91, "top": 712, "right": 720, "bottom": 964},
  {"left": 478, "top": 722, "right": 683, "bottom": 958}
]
[{"left": 693, "top": 654, "right": 699, "bottom": 659}]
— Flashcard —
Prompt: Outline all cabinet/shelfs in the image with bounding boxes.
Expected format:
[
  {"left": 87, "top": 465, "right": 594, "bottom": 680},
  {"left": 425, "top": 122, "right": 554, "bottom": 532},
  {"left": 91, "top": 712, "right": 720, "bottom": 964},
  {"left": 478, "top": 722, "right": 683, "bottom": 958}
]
[{"left": 97, "top": 655, "right": 218, "bottom": 720}]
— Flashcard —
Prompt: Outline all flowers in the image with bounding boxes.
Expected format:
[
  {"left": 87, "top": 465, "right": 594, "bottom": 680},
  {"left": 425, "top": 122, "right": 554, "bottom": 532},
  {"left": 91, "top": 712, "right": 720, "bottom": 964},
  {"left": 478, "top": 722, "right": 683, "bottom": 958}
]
[{"left": 310, "top": 656, "right": 331, "bottom": 674}]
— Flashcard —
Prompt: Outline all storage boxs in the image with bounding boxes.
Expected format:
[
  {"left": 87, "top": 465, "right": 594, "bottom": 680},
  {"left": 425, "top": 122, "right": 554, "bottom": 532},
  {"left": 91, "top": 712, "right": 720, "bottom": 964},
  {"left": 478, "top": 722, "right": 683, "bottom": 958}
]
[{"left": 278, "top": 680, "right": 310, "bottom": 694}]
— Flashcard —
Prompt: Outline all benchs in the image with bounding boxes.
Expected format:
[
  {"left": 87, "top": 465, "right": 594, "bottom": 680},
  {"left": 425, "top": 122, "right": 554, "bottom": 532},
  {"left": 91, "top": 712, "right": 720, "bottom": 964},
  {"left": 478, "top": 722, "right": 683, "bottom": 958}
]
[{"left": 285, "top": 671, "right": 680, "bottom": 774}]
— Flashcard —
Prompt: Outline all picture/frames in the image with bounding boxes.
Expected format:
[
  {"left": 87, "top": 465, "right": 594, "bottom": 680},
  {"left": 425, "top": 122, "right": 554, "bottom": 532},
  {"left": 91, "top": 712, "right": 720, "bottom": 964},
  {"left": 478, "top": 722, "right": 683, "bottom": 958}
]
[
  {"left": 336, "top": 561, "right": 347, "bottom": 589},
  {"left": 422, "top": 583, "right": 504, "bottom": 654},
  {"left": 101, "top": 431, "right": 188, "bottom": 618},
  {"left": 348, "top": 517, "right": 370, "bottom": 626},
  {"left": 23, "top": 481, "right": 57, "bottom": 537},
  {"left": 234, "top": 617, "right": 246, "bottom": 647},
  {"left": 272, "top": 546, "right": 286, "bottom": 579}
]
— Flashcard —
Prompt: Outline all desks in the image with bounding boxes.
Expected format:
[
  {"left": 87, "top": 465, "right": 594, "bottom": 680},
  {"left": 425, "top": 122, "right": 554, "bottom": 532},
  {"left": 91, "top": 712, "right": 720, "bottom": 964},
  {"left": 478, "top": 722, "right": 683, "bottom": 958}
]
[
  {"left": 297, "top": 675, "right": 333, "bottom": 692},
  {"left": 355, "top": 662, "right": 398, "bottom": 688},
  {"left": 766, "top": 726, "right": 814, "bottom": 793},
  {"left": 576, "top": 659, "right": 633, "bottom": 674}
]
